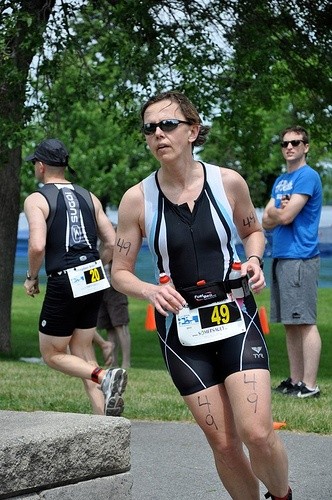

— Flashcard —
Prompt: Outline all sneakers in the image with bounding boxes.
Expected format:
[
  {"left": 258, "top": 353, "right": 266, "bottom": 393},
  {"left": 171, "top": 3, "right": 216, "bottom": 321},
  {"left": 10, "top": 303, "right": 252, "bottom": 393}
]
[
  {"left": 97, "top": 368, "right": 128, "bottom": 416},
  {"left": 284, "top": 381, "right": 320, "bottom": 399},
  {"left": 274, "top": 377, "right": 294, "bottom": 393}
]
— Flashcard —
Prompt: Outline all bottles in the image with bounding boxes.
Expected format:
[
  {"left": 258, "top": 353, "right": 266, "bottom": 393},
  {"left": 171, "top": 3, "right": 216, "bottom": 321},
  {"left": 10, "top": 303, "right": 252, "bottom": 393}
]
[
  {"left": 158, "top": 272, "right": 176, "bottom": 290},
  {"left": 229, "top": 261, "right": 245, "bottom": 299}
]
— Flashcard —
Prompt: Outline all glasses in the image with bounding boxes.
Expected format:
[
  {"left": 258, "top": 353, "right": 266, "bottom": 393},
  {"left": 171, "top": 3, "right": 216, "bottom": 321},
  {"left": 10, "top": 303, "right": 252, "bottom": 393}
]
[
  {"left": 31, "top": 159, "right": 40, "bottom": 165},
  {"left": 144, "top": 119, "right": 193, "bottom": 135},
  {"left": 281, "top": 140, "right": 304, "bottom": 147}
]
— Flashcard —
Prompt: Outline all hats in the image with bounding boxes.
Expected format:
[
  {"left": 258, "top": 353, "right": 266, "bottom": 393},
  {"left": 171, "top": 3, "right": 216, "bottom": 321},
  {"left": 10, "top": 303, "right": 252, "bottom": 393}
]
[{"left": 25, "top": 139, "right": 69, "bottom": 166}]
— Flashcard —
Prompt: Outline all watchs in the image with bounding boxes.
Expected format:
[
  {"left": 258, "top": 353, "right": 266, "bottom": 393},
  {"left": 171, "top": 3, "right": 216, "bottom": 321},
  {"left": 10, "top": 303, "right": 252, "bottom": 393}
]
[
  {"left": 247, "top": 256, "right": 264, "bottom": 269},
  {"left": 26, "top": 271, "right": 38, "bottom": 281}
]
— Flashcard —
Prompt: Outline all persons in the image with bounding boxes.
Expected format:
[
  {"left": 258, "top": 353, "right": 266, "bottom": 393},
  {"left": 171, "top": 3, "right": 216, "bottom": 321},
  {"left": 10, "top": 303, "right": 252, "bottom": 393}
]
[
  {"left": 24, "top": 138, "right": 130, "bottom": 416},
  {"left": 111, "top": 90, "right": 293, "bottom": 500},
  {"left": 262, "top": 127, "right": 322, "bottom": 398}
]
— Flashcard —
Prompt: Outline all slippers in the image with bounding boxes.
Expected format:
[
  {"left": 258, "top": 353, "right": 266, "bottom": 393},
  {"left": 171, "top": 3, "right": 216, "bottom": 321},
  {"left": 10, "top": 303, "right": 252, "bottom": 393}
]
[{"left": 103, "top": 342, "right": 115, "bottom": 367}]
